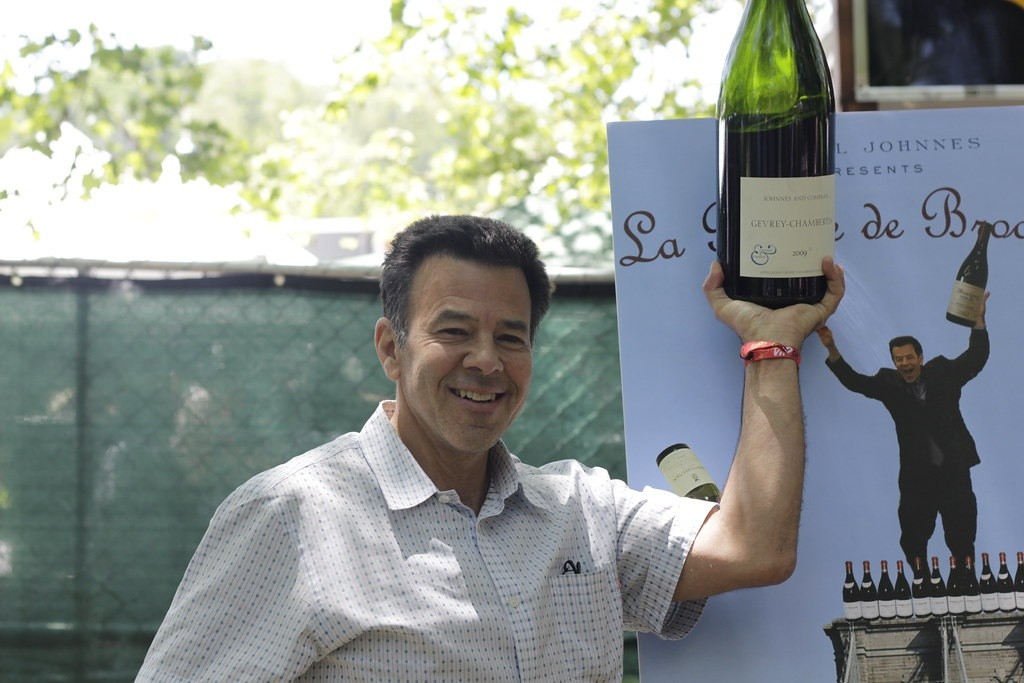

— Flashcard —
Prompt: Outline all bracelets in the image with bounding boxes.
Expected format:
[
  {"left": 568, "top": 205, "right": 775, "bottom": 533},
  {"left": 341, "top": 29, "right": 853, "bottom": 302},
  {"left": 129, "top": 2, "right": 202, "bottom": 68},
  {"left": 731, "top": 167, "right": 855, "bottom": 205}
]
[{"left": 740, "top": 341, "right": 800, "bottom": 367}]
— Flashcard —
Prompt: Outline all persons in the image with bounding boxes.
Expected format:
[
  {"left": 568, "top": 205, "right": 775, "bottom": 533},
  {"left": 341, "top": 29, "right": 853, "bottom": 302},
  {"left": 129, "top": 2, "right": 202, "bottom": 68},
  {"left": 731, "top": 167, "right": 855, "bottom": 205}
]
[
  {"left": 134, "top": 216, "right": 845, "bottom": 683},
  {"left": 817, "top": 292, "right": 990, "bottom": 584}
]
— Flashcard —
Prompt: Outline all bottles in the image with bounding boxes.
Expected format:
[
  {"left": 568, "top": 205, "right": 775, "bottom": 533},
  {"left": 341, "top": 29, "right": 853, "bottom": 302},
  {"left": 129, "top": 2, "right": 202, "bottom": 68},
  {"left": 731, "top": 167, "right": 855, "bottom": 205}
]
[
  {"left": 946, "top": 556, "right": 965, "bottom": 615},
  {"left": 1014, "top": 552, "right": 1024, "bottom": 611},
  {"left": 859, "top": 560, "right": 879, "bottom": 620},
  {"left": 945, "top": 222, "right": 994, "bottom": 327},
  {"left": 716, "top": 0, "right": 838, "bottom": 307},
  {"left": 841, "top": 561, "right": 862, "bottom": 620},
  {"left": 964, "top": 556, "right": 982, "bottom": 614},
  {"left": 997, "top": 552, "right": 1016, "bottom": 612},
  {"left": 655, "top": 443, "right": 722, "bottom": 502},
  {"left": 895, "top": 560, "right": 913, "bottom": 618},
  {"left": 911, "top": 556, "right": 931, "bottom": 618},
  {"left": 929, "top": 556, "right": 948, "bottom": 617},
  {"left": 979, "top": 552, "right": 1000, "bottom": 613},
  {"left": 877, "top": 560, "right": 896, "bottom": 619}
]
